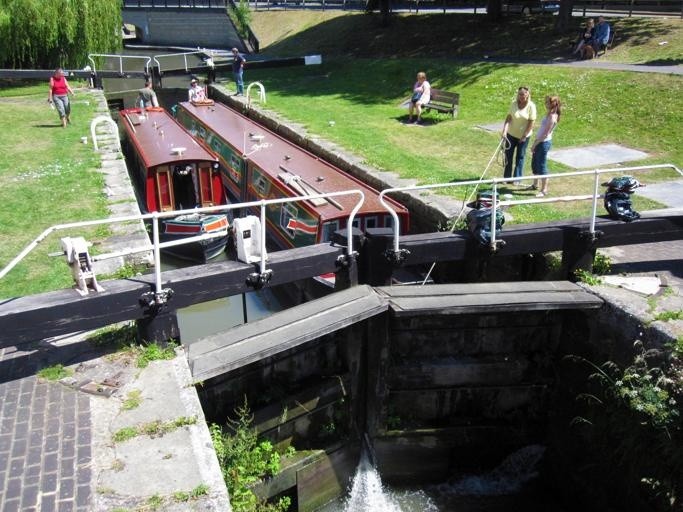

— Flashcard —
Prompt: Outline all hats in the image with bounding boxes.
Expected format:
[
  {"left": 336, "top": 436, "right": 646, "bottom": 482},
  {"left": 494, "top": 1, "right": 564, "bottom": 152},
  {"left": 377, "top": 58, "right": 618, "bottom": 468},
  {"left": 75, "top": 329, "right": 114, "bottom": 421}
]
[{"left": 189, "top": 78, "right": 198, "bottom": 83}]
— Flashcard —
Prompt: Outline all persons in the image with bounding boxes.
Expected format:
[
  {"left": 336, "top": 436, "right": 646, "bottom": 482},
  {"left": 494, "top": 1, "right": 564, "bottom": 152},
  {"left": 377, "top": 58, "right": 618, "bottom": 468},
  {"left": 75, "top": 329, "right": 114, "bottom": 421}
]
[
  {"left": 502, "top": 86, "right": 537, "bottom": 185},
  {"left": 570, "top": 17, "right": 595, "bottom": 60},
  {"left": 188, "top": 79, "right": 206, "bottom": 103},
  {"left": 47, "top": 66, "right": 76, "bottom": 127},
  {"left": 407, "top": 72, "right": 430, "bottom": 124},
  {"left": 581, "top": 17, "right": 611, "bottom": 61},
  {"left": 232, "top": 48, "right": 246, "bottom": 96},
  {"left": 523, "top": 93, "right": 561, "bottom": 197},
  {"left": 135, "top": 82, "right": 159, "bottom": 110}
]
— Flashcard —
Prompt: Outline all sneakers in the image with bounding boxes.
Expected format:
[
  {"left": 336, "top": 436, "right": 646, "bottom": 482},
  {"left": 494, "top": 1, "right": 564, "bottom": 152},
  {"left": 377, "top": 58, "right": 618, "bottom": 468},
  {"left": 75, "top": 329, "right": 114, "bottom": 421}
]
[
  {"left": 535, "top": 189, "right": 547, "bottom": 197},
  {"left": 527, "top": 185, "right": 539, "bottom": 190}
]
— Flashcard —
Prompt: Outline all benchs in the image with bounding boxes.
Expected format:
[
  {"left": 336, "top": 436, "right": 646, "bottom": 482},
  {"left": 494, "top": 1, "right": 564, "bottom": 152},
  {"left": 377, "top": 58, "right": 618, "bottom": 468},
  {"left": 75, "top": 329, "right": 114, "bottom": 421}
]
[
  {"left": 570, "top": 28, "right": 617, "bottom": 58},
  {"left": 425, "top": 89, "right": 459, "bottom": 118}
]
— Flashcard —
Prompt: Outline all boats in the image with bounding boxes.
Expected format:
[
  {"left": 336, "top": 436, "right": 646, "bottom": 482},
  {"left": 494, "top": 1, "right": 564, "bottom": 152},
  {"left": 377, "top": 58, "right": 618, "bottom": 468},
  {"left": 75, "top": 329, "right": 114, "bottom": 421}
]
[
  {"left": 118, "top": 105, "right": 233, "bottom": 265},
  {"left": 173, "top": 96, "right": 410, "bottom": 305}
]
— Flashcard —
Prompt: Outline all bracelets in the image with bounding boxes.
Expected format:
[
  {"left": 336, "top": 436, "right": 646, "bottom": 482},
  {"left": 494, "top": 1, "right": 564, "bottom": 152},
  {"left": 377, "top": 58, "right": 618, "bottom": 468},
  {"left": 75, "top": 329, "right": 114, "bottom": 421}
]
[{"left": 522, "top": 135, "right": 527, "bottom": 138}]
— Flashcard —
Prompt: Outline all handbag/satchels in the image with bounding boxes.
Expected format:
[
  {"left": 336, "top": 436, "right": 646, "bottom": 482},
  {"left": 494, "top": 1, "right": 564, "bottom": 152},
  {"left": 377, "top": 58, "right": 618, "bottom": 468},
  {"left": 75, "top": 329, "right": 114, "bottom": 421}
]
[{"left": 412, "top": 91, "right": 421, "bottom": 102}]
